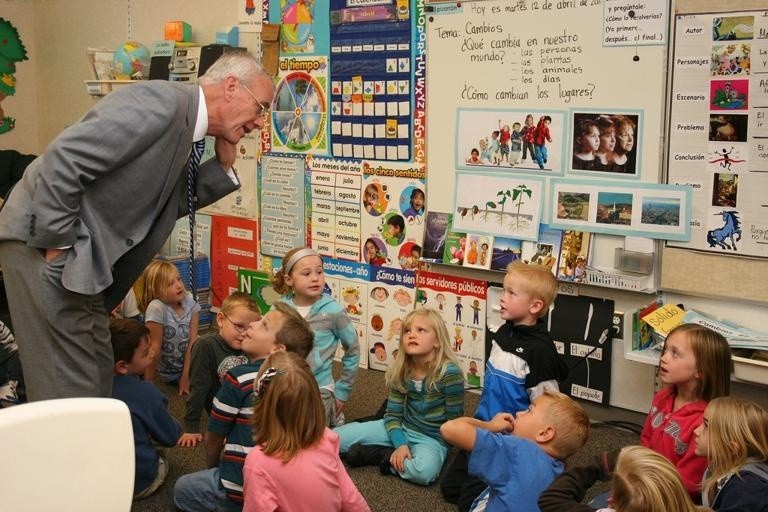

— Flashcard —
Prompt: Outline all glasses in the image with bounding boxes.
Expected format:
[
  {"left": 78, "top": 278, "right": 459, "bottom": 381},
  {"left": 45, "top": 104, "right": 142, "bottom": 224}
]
[
  {"left": 225, "top": 316, "right": 245, "bottom": 333},
  {"left": 243, "top": 84, "right": 268, "bottom": 116}
]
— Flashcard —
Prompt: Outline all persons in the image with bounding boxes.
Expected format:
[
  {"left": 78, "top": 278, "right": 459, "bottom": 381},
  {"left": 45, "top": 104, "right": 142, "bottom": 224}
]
[
  {"left": 1, "top": 51, "right": 276, "bottom": 401},
  {"left": 465, "top": 113, "right": 553, "bottom": 170},
  {"left": 403, "top": 189, "right": 424, "bottom": 219},
  {"left": 383, "top": 215, "right": 405, "bottom": 244},
  {"left": 364, "top": 239, "right": 384, "bottom": 266},
  {"left": 363, "top": 184, "right": 379, "bottom": 216},
  {"left": 573, "top": 115, "right": 637, "bottom": 172},
  {"left": 1, "top": 247, "right": 767, "bottom": 512}
]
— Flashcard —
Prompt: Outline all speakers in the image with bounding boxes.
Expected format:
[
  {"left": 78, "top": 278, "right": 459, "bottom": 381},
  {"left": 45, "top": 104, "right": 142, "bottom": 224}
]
[
  {"left": 149, "top": 56, "right": 171, "bottom": 80},
  {"left": 197, "top": 44, "right": 247, "bottom": 77}
]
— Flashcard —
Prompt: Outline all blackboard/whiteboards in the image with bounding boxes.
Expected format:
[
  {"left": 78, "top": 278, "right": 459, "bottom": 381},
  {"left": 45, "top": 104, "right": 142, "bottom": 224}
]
[{"left": 418, "top": 1, "right": 675, "bottom": 296}]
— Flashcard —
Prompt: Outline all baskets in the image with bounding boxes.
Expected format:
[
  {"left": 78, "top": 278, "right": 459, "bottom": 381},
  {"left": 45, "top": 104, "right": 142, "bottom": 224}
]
[
  {"left": 584, "top": 265, "right": 650, "bottom": 291},
  {"left": 614, "top": 247, "right": 656, "bottom": 274}
]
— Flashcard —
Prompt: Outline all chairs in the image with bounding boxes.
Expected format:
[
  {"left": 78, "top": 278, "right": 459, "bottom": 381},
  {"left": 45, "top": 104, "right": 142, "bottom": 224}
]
[{"left": 0, "top": 397, "right": 137, "bottom": 511}]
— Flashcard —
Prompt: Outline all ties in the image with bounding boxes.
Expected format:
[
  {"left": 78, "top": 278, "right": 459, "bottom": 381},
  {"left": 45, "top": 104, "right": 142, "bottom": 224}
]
[{"left": 188, "top": 137, "right": 206, "bottom": 300}]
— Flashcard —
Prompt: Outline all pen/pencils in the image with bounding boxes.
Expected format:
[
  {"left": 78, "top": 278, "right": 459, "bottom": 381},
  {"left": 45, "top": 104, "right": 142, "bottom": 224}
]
[{"left": 585, "top": 266, "right": 603, "bottom": 273}]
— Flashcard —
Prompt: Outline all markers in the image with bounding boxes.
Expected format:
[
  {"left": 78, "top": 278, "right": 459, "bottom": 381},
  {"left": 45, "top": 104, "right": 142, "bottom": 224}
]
[{"left": 419, "top": 256, "right": 443, "bottom": 263}]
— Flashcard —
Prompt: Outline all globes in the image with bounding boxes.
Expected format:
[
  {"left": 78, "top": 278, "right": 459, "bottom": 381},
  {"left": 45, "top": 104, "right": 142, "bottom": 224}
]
[{"left": 113, "top": 41, "right": 150, "bottom": 75}]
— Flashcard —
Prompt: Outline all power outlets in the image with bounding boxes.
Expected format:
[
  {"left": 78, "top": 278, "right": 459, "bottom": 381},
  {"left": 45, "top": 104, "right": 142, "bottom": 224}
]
[{"left": 611, "top": 311, "right": 623, "bottom": 340}]
